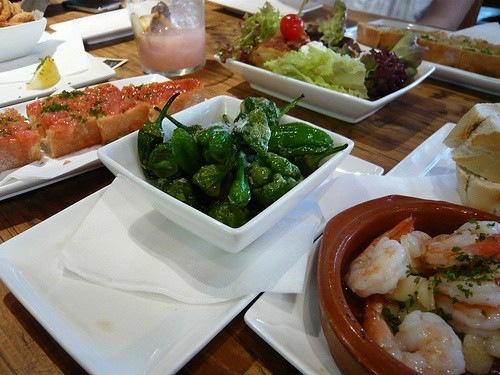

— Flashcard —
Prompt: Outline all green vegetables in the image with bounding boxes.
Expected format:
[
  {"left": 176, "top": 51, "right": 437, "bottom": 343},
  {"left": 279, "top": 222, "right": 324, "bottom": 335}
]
[
  {"left": 32, "top": 55, "right": 54, "bottom": 76},
  {"left": 380, "top": 216, "right": 500, "bottom": 336},
  {"left": 0, "top": 87, "right": 151, "bottom": 138},
  {"left": 216, "top": 0, "right": 429, "bottom": 101}
]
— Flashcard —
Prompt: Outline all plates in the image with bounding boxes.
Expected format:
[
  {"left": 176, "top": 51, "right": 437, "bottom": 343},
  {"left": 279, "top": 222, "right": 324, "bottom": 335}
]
[
  {"left": 243, "top": 120, "right": 461, "bottom": 375},
  {"left": 0, "top": 45, "right": 116, "bottom": 108},
  {"left": 206, "top": 0, "right": 324, "bottom": 19},
  {"left": 214, "top": 39, "right": 435, "bottom": 123},
  {"left": 344, "top": 17, "right": 500, "bottom": 98},
  {"left": 0, "top": 73, "right": 207, "bottom": 201},
  {"left": 0, "top": 153, "right": 385, "bottom": 375}
]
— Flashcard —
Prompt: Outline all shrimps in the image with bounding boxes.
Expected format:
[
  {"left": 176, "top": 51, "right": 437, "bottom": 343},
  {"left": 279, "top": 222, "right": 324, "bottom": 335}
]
[{"left": 344, "top": 212, "right": 500, "bottom": 375}]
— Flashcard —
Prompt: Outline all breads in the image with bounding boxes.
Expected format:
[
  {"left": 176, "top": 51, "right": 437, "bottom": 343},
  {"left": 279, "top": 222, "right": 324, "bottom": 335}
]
[
  {"left": 442, "top": 101, "right": 500, "bottom": 220},
  {"left": 0, "top": 0, "right": 35, "bottom": 29},
  {"left": 356, "top": 22, "right": 500, "bottom": 81}
]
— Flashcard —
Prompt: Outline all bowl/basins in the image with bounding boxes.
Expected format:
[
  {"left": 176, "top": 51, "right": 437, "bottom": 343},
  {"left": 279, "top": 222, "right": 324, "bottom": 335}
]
[
  {"left": 317, "top": 194, "right": 500, "bottom": 375},
  {"left": 96, "top": 94, "right": 354, "bottom": 256},
  {"left": 0, "top": 16, "right": 47, "bottom": 63}
]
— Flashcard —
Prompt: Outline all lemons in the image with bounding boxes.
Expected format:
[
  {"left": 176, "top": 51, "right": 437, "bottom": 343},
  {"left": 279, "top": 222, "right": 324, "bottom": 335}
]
[{"left": 28, "top": 57, "right": 60, "bottom": 89}]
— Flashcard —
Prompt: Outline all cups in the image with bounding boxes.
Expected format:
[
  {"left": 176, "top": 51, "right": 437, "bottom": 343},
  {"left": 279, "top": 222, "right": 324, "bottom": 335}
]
[{"left": 126, "top": 0, "right": 206, "bottom": 77}]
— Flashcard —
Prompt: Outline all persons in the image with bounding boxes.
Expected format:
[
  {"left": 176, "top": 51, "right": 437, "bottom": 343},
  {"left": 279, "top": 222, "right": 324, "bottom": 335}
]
[{"left": 321, "top": 0, "right": 475, "bottom": 32}]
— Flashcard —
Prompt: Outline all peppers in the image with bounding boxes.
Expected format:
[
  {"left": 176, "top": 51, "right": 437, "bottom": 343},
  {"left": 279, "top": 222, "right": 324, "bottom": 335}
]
[{"left": 137, "top": 90, "right": 348, "bottom": 227}]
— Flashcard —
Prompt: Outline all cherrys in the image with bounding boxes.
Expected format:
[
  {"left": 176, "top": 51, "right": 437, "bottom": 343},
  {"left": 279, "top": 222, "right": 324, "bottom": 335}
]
[{"left": 280, "top": 14, "right": 305, "bottom": 39}]
